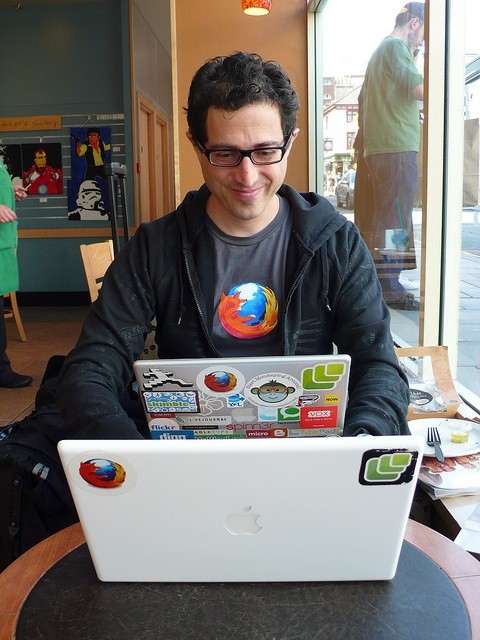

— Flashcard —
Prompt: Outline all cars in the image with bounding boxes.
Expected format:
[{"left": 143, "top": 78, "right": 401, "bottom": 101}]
[{"left": 335, "top": 169, "right": 355, "bottom": 209}]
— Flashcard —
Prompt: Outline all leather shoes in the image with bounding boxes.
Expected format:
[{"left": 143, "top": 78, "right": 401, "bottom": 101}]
[
  {"left": 0, "top": 366, "right": 33, "bottom": 388},
  {"left": 387, "top": 293, "right": 420, "bottom": 311}
]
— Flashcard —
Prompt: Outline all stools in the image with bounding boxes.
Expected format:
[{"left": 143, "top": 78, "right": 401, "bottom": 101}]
[{"left": 2, "top": 290, "right": 27, "bottom": 340}]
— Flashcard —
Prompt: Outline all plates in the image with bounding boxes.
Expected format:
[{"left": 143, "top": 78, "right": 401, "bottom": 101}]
[{"left": 406, "top": 417, "right": 480, "bottom": 458}]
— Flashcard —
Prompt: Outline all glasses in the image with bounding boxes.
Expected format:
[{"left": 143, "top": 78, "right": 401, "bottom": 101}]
[{"left": 189, "top": 130, "right": 292, "bottom": 167}]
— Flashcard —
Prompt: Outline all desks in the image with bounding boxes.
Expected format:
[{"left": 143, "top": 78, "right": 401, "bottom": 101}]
[{"left": 0, "top": 514, "right": 480, "bottom": 639}]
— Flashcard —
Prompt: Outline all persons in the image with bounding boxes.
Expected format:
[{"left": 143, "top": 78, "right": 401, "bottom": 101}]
[
  {"left": 69, "top": 128, "right": 112, "bottom": 181},
  {"left": 364, "top": 1, "right": 424, "bottom": 312},
  {"left": 57, "top": 52, "right": 410, "bottom": 440},
  {"left": 352, "top": 84, "right": 373, "bottom": 248},
  {"left": 0, "top": 159, "right": 33, "bottom": 389}
]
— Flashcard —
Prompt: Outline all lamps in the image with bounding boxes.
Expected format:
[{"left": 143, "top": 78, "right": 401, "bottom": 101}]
[{"left": 242, "top": 0, "right": 271, "bottom": 16}]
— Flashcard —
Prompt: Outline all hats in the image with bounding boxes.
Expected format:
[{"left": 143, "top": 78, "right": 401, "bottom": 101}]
[{"left": 400, "top": 2, "right": 424, "bottom": 20}]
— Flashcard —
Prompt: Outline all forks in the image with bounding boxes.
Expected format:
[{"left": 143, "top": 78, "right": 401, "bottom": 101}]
[{"left": 428, "top": 427, "right": 445, "bottom": 463}]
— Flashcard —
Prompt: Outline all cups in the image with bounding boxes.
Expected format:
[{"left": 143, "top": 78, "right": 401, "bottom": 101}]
[{"left": 385, "top": 229, "right": 403, "bottom": 250}]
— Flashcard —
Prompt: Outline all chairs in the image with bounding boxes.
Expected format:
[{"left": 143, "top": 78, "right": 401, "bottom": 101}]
[{"left": 78, "top": 239, "right": 116, "bottom": 303}]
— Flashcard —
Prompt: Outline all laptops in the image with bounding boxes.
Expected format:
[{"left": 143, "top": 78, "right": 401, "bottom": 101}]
[
  {"left": 54, "top": 436, "right": 431, "bottom": 583},
  {"left": 127, "top": 353, "right": 354, "bottom": 438}
]
